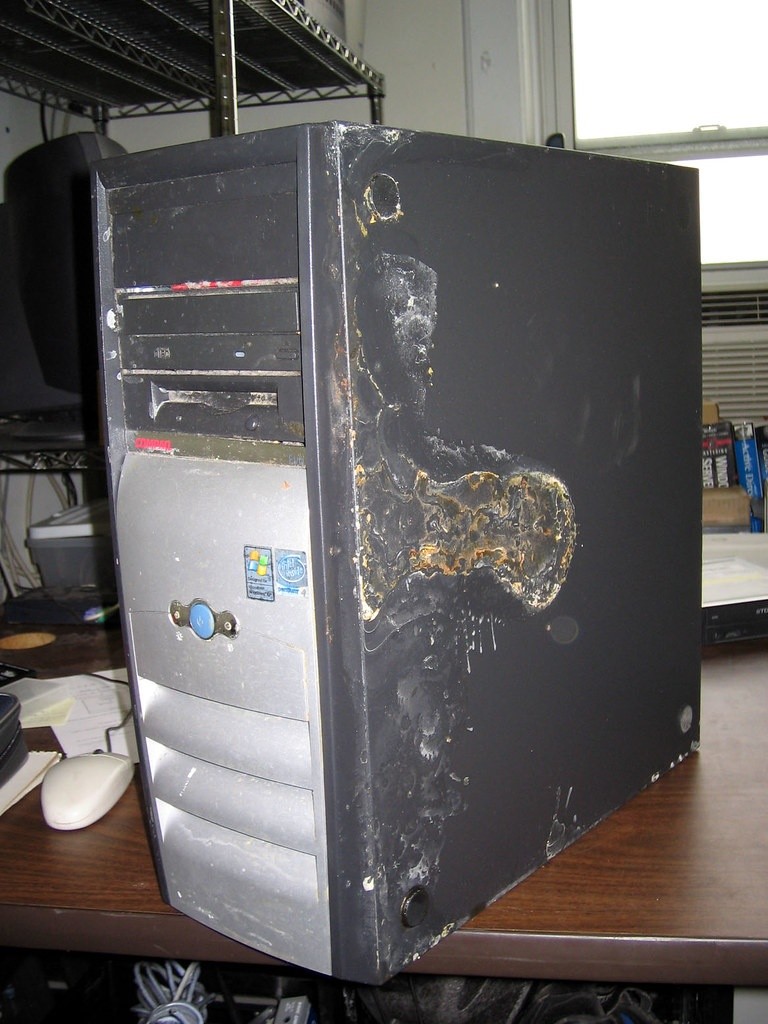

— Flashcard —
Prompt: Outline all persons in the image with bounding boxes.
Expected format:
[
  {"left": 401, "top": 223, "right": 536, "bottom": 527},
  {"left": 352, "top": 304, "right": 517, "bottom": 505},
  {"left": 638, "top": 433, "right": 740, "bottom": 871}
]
[{"left": 736, "top": 427, "right": 750, "bottom": 438}]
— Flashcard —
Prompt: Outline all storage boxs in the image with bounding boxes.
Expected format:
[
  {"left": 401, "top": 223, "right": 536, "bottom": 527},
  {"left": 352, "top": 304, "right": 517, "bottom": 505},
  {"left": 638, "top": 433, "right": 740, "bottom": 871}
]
[
  {"left": 700, "top": 484, "right": 751, "bottom": 533},
  {"left": 23, "top": 493, "right": 116, "bottom": 604}
]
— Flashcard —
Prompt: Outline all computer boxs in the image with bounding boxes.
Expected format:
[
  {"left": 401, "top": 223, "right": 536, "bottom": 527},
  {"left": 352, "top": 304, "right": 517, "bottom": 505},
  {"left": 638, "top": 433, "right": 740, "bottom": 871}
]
[{"left": 87, "top": 119, "right": 704, "bottom": 988}]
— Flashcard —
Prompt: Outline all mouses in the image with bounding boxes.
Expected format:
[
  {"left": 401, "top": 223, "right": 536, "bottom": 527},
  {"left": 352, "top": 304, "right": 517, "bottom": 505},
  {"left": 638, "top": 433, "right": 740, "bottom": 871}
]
[{"left": 41, "top": 749, "right": 136, "bottom": 830}]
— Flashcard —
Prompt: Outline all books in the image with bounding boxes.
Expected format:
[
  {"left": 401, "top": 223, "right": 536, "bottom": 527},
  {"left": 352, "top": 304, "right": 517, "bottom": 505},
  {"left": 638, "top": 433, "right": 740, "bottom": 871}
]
[{"left": 702, "top": 422, "right": 768, "bottom": 532}]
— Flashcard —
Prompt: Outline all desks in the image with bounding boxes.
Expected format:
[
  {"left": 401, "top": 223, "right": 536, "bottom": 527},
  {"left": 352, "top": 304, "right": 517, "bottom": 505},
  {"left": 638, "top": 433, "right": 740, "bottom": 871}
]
[{"left": 0, "top": 622, "right": 768, "bottom": 987}]
